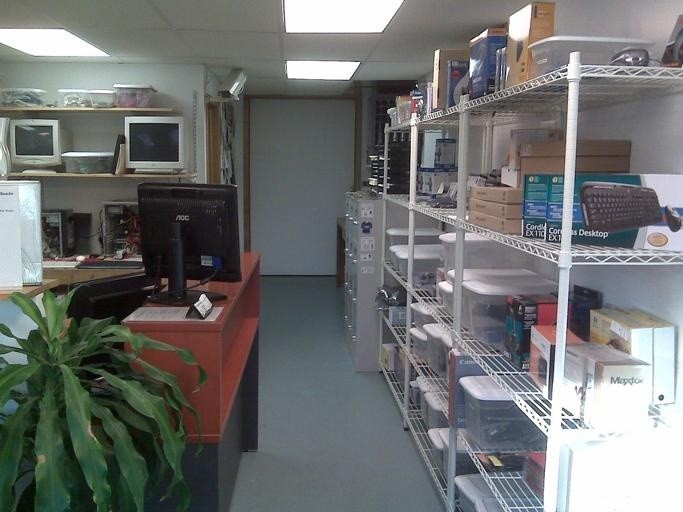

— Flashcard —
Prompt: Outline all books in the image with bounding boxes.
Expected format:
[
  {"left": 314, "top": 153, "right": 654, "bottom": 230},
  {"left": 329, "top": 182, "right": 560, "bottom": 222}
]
[{"left": 77, "top": 260, "right": 143, "bottom": 270}]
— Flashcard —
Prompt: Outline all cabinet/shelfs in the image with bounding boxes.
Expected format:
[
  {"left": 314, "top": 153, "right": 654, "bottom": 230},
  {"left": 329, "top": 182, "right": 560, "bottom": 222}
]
[
  {"left": 0, "top": 106, "right": 197, "bottom": 178},
  {"left": 344, "top": 191, "right": 438, "bottom": 373}
]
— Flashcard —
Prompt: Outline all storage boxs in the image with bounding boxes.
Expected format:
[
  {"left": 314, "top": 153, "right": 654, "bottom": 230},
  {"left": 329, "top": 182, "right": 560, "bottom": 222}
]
[
  {"left": 61, "top": 152, "right": 115, "bottom": 174},
  {"left": 116, "top": 87, "right": 157, "bottom": 107},
  {"left": 90, "top": 93, "right": 118, "bottom": 107}
]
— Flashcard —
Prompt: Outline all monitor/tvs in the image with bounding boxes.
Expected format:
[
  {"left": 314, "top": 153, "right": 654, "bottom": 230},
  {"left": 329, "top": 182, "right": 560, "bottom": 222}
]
[
  {"left": 66, "top": 272, "right": 161, "bottom": 329},
  {"left": 137, "top": 182, "right": 243, "bottom": 306},
  {"left": 125, "top": 116, "right": 186, "bottom": 174},
  {"left": 10, "top": 120, "right": 72, "bottom": 174}
]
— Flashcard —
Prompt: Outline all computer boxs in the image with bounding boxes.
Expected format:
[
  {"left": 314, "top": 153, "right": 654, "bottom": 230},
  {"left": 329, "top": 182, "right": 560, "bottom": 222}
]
[
  {"left": 41, "top": 208, "right": 76, "bottom": 258},
  {"left": 101, "top": 202, "right": 143, "bottom": 259}
]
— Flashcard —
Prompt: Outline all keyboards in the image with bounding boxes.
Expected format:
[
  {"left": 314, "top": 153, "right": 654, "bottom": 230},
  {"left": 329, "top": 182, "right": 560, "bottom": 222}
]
[
  {"left": 76, "top": 261, "right": 142, "bottom": 269},
  {"left": 581, "top": 183, "right": 663, "bottom": 233}
]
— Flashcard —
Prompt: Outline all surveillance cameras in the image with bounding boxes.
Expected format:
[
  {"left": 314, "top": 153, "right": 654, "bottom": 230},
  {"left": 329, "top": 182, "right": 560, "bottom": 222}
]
[{"left": 218, "top": 70, "right": 248, "bottom": 102}]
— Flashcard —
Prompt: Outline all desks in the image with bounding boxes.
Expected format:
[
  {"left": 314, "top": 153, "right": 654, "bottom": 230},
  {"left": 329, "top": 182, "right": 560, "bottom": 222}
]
[
  {"left": 120, "top": 252, "right": 261, "bottom": 512},
  {"left": 336, "top": 217, "right": 345, "bottom": 288}
]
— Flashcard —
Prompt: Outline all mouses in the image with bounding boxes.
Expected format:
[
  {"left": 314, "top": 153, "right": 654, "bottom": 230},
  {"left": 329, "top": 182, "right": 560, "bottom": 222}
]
[
  {"left": 609, "top": 47, "right": 649, "bottom": 66},
  {"left": 75, "top": 256, "right": 86, "bottom": 261},
  {"left": 665, "top": 205, "right": 681, "bottom": 232}
]
